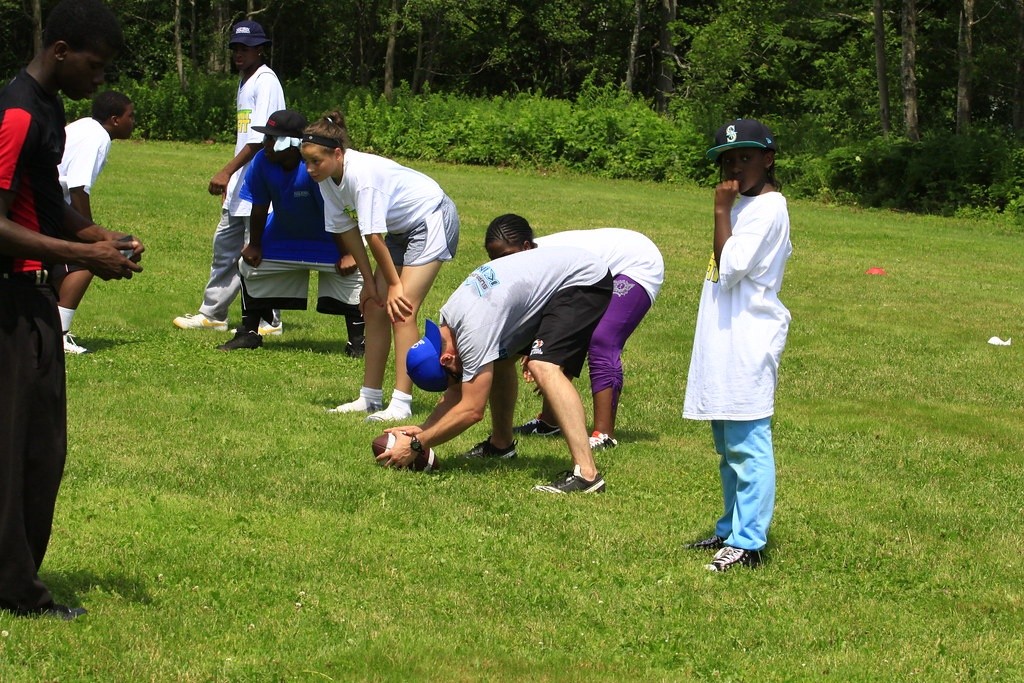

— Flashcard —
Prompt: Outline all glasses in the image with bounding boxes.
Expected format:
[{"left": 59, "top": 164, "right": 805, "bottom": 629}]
[{"left": 446, "top": 367, "right": 463, "bottom": 385}]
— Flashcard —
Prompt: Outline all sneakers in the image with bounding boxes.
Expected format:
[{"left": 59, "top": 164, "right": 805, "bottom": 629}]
[
  {"left": 513, "top": 418, "right": 561, "bottom": 438},
  {"left": 463, "top": 436, "right": 518, "bottom": 460},
  {"left": 685, "top": 531, "right": 724, "bottom": 551},
  {"left": 346, "top": 340, "right": 366, "bottom": 358},
  {"left": 231, "top": 317, "right": 285, "bottom": 335},
  {"left": 703, "top": 544, "right": 759, "bottom": 573},
  {"left": 38, "top": 604, "right": 88, "bottom": 623},
  {"left": 172, "top": 309, "right": 229, "bottom": 333},
  {"left": 367, "top": 404, "right": 411, "bottom": 421},
  {"left": 588, "top": 431, "right": 616, "bottom": 449},
  {"left": 215, "top": 331, "right": 263, "bottom": 351},
  {"left": 63, "top": 332, "right": 87, "bottom": 355},
  {"left": 535, "top": 469, "right": 606, "bottom": 496},
  {"left": 330, "top": 400, "right": 383, "bottom": 414}
]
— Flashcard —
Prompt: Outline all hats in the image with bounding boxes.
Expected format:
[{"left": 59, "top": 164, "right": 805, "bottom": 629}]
[
  {"left": 405, "top": 320, "right": 447, "bottom": 392},
  {"left": 251, "top": 110, "right": 307, "bottom": 138},
  {"left": 227, "top": 21, "right": 270, "bottom": 48},
  {"left": 706, "top": 121, "right": 774, "bottom": 163}
]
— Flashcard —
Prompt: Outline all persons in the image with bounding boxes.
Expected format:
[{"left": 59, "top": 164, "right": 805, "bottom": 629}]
[
  {"left": 171, "top": 22, "right": 290, "bottom": 336},
  {"left": 485, "top": 214, "right": 665, "bottom": 449},
  {"left": 0, "top": 0, "right": 145, "bottom": 621},
  {"left": 376, "top": 245, "right": 614, "bottom": 496},
  {"left": 301, "top": 111, "right": 459, "bottom": 422},
  {"left": 216, "top": 110, "right": 366, "bottom": 358},
  {"left": 681, "top": 125, "right": 793, "bottom": 572},
  {"left": 58, "top": 91, "right": 135, "bottom": 353}
]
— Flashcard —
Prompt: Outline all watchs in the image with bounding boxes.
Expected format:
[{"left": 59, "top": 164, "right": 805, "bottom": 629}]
[{"left": 410, "top": 436, "right": 424, "bottom": 454}]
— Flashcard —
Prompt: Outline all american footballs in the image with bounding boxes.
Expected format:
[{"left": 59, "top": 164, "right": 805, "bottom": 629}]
[{"left": 370, "top": 432, "right": 440, "bottom": 474}]
